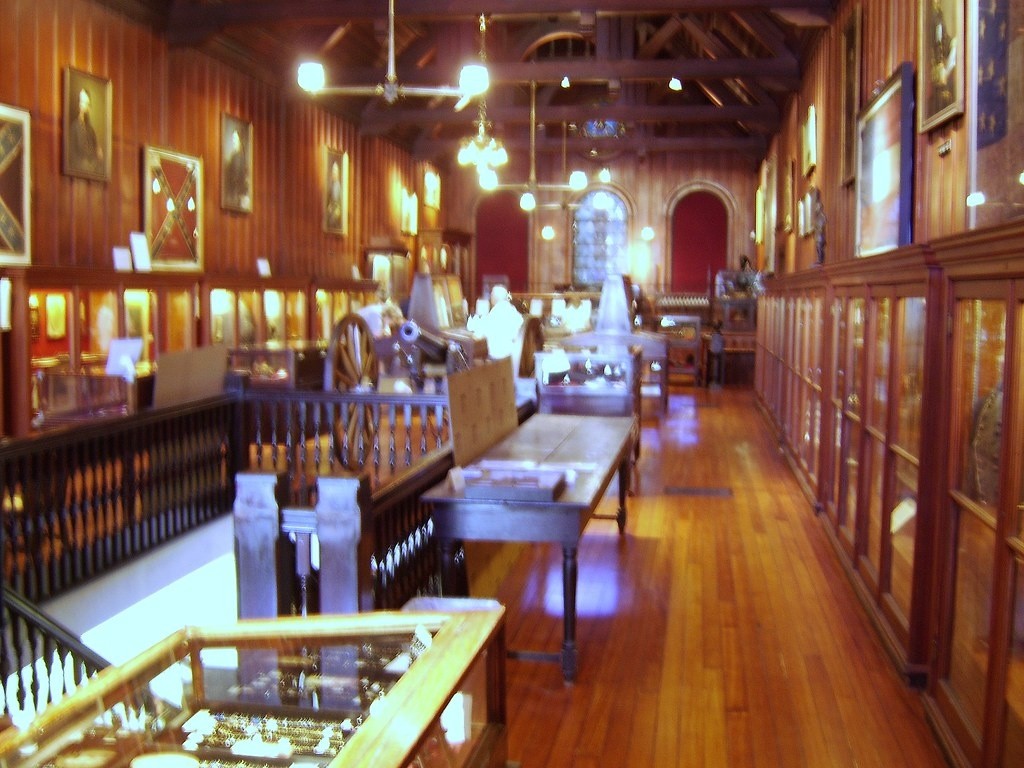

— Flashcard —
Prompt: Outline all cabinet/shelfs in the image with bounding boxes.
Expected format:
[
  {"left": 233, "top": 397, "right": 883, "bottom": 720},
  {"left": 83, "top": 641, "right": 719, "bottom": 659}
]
[
  {"left": 3, "top": 267, "right": 380, "bottom": 437},
  {"left": 362, "top": 227, "right": 471, "bottom": 313},
  {"left": 753, "top": 217, "right": 1023, "bottom": 767}
]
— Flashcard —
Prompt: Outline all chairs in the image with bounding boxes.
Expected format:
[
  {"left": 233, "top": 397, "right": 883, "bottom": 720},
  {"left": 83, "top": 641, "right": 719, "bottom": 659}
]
[{"left": 621, "top": 274, "right": 702, "bottom": 386}]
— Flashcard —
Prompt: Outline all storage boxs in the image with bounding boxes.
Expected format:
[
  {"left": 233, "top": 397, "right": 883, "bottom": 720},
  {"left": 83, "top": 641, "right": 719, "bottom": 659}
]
[{"left": 464, "top": 469, "right": 566, "bottom": 503}]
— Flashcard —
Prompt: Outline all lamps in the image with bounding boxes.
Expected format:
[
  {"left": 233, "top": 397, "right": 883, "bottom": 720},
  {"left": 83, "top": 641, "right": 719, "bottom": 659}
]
[
  {"left": 298, "top": 0, "right": 491, "bottom": 102},
  {"left": 458, "top": 12, "right": 508, "bottom": 171},
  {"left": 479, "top": 83, "right": 608, "bottom": 210}
]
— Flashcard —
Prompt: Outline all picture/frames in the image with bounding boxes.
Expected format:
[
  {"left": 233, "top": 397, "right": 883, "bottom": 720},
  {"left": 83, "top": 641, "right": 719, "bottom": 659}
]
[
  {"left": 142, "top": 142, "right": 204, "bottom": 272},
  {"left": 220, "top": 111, "right": 254, "bottom": 214},
  {"left": 852, "top": 62, "right": 914, "bottom": 259},
  {"left": 63, "top": 64, "right": 114, "bottom": 183},
  {"left": 0, "top": 103, "right": 31, "bottom": 267},
  {"left": 796, "top": 186, "right": 819, "bottom": 237},
  {"left": 801, "top": 105, "right": 816, "bottom": 179},
  {"left": 914, "top": 0, "right": 965, "bottom": 134},
  {"left": 322, "top": 146, "right": 349, "bottom": 234}
]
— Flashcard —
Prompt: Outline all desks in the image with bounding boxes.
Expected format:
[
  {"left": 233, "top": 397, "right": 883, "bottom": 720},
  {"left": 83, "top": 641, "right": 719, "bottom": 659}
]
[
  {"left": 701, "top": 328, "right": 757, "bottom": 387},
  {"left": 421, "top": 414, "right": 638, "bottom": 681},
  {"left": 717, "top": 299, "right": 755, "bottom": 330}
]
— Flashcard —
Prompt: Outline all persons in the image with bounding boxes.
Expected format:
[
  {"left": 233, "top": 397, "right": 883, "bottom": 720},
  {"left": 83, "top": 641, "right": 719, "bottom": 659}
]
[{"left": 472, "top": 284, "right": 523, "bottom": 362}]
